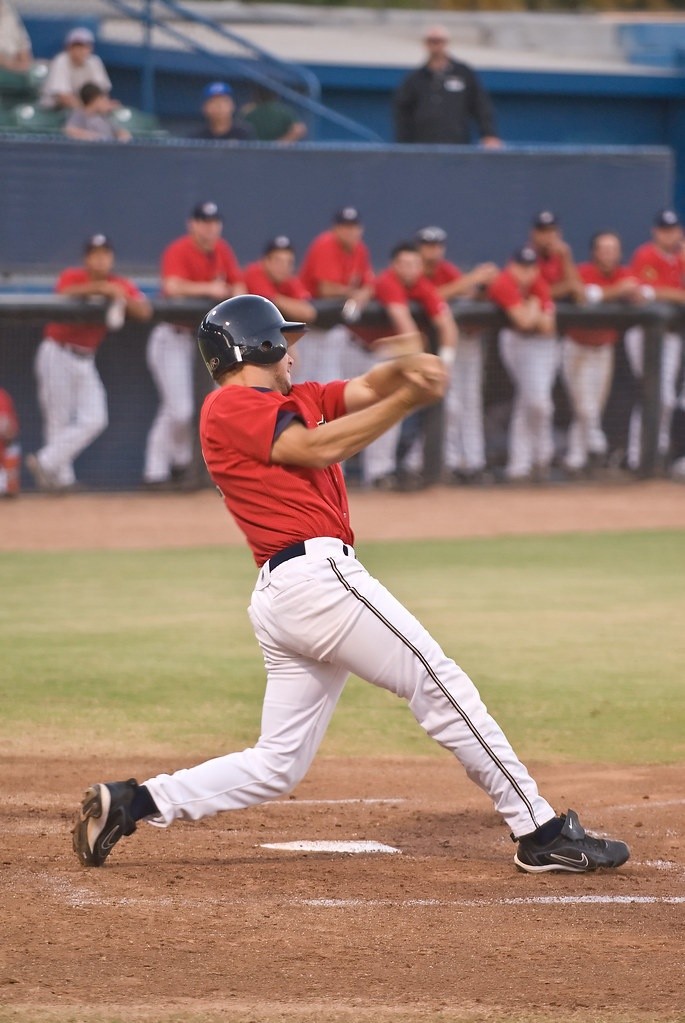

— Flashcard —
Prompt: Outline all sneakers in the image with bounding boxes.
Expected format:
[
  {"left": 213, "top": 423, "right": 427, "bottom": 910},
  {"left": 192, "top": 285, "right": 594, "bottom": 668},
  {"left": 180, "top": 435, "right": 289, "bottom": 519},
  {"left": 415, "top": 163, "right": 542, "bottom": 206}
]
[
  {"left": 513, "top": 809, "right": 631, "bottom": 875},
  {"left": 70, "top": 777, "right": 139, "bottom": 868}
]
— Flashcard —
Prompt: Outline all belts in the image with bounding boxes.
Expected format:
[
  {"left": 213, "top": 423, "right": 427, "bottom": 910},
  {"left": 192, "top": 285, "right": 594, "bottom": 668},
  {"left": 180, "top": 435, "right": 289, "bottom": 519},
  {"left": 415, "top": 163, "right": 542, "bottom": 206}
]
[{"left": 262, "top": 543, "right": 356, "bottom": 579}]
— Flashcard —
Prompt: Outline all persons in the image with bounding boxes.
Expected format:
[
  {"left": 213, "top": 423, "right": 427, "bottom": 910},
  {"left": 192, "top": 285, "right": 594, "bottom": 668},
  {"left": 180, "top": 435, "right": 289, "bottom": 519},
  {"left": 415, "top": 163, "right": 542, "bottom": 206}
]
[
  {"left": 69, "top": 292, "right": 631, "bottom": 874},
  {"left": 143, "top": 203, "right": 248, "bottom": 491},
  {"left": 619, "top": 209, "right": 685, "bottom": 475},
  {"left": 0, "top": 391, "right": 22, "bottom": 498},
  {"left": 227, "top": 79, "right": 308, "bottom": 142},
  {"left": 0, "top": 0, "right": 37, "bottom": 77},
  {"left": 181, "top": 82, "right": 259, "bottom": 142},
  {"left": 396, "top": 23, "right": 502, "bottom": 147},
  {"left": 440, "top": 209, "right": 590, "bottom": 484},
  {"left": 23, "top": 234, "right": 152, "bottom": 495},
  {"left": 34, "top": 25, "right": 111, "bottom": 112},
  {"left": 292, "top": 208, "right": 497, "bottom": 493},
  {"left": 557, "top": 232, "right": 641, "bottom": 477},
  {"left": 243, "top": 235, "right": 318, "bottom": 347},
  {"left": 62, "top": 82, "right": 134, "bottom": 142}
]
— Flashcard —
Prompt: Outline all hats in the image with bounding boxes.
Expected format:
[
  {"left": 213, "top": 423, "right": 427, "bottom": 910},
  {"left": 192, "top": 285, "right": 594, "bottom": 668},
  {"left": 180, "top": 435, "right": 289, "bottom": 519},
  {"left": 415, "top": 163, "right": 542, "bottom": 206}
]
[
  {"left": 263, "top": 235, "right": 293, "bottom": 254},
  {"left": 200, "top": 81, "right": 233, "bottom": 100},
  {"left": 417, "top": 226, "right": 447, "bottom": 245},
  {"left": 83, "top": 234, "right": 113, "bottom": 254},
  {"left": 510, "top": 245, "right": 537, "bottom": 266},
  {"left": 534, "top": 212, "right": 561, "bottom": 230},
  {"left": 654, "top": 208, "right": 680, "bottom": 228},
  {"left": 64, "top": 26, "right": 95, "bottom": 44},
  {"left": 334, "top": 206, "right": 363, "bottom": 225},
  {"left": 195, "top": 198, "right": 222, "bottom": 221}
]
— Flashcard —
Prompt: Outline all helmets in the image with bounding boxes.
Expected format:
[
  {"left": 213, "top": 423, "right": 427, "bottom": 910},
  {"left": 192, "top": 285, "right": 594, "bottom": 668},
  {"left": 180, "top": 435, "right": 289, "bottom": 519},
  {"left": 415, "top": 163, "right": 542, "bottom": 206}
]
[{"left": 197, "top": 294, "right": 307, "bottom": 380}]
